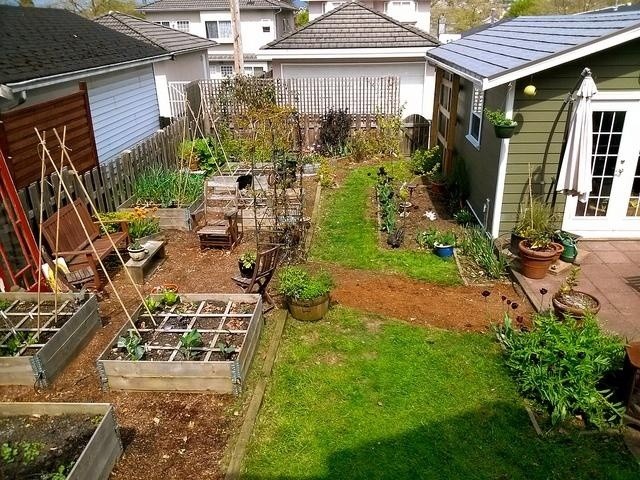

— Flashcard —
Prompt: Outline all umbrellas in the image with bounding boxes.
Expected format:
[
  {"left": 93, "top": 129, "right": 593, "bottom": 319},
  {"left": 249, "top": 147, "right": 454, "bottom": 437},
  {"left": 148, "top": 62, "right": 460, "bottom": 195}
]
[{"left": 539, "top": 68, "right": 598, "bottom": 231}]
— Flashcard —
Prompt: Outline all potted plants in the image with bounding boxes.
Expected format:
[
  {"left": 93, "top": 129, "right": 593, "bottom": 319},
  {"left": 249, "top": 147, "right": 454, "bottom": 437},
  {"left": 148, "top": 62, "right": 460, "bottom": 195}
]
[
  {"left": 127, "top": 241, "right": 147, "bottom": 261},
  {"left": 552, "top": 264, "right": 601, "bottom": 327},
  {"left": 483, "top": 106, "right": 517, "bottom": 137},
  {"left": 509, "top": 202, "right": 564, "bottom": 279},
  {"left": 433, "top": 231, "right": 458, "bottom": 258},
  {"left": 278, "top": 262, "right": 337, "bottom": 322},
  {"left": 430, "top": 167, "right": 448, "bottom": 197},
  {"left": 237, "top": 251, "right": 256, "bottom": 279}
]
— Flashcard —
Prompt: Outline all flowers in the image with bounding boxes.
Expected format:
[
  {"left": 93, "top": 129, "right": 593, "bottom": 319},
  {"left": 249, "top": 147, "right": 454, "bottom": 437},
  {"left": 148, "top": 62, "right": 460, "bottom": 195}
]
[{"left": 92, "top": 204, "right": 162, "bottom": 239}]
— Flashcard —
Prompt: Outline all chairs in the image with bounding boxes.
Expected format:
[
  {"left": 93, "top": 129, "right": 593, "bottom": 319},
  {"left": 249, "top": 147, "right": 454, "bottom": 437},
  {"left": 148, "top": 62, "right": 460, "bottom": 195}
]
[
  {"left": 228, "top": 245, "right": 282, "bottom": 313},
  {"left": 189, "top": 179, "right": 243, "bottom": 254}
]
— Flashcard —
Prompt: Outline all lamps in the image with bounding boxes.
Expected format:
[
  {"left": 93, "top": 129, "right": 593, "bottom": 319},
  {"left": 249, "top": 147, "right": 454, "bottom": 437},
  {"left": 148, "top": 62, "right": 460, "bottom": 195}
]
[{"left": 524, "top": 75, "right": 538, "bottom": 98}]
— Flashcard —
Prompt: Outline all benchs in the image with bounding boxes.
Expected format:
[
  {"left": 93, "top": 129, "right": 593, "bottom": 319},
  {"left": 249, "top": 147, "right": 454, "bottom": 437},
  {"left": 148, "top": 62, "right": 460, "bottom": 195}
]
[{"left": 38, "top": 195, "right": 132, "bottom": 293}]
